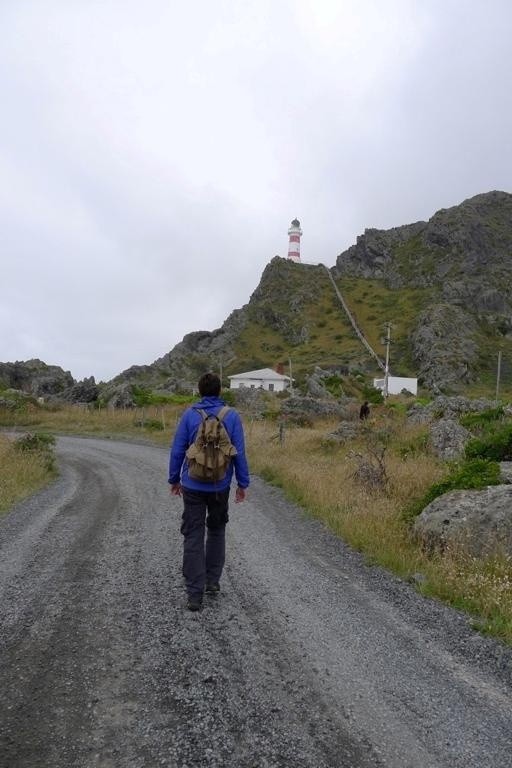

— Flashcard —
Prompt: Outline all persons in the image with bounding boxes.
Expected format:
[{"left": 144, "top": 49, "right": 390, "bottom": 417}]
[
  {"left": 168, "top": 372, "right": 250, "bottom": 611},
  {"left": 359, "top": 401, "right": 370, "bottom": 421}
]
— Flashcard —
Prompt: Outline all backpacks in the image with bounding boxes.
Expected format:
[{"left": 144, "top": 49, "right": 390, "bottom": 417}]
[{"left": 184, "top": 406, "right": 237, "bottom": 482}]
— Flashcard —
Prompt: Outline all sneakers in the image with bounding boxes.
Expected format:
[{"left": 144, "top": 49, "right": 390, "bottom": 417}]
[
  {"left": 205, "top": 580, "right": 221, "bottom": 592},
  {"left": 187, "top": 593, "right": 203, "bottom": 610}
]
